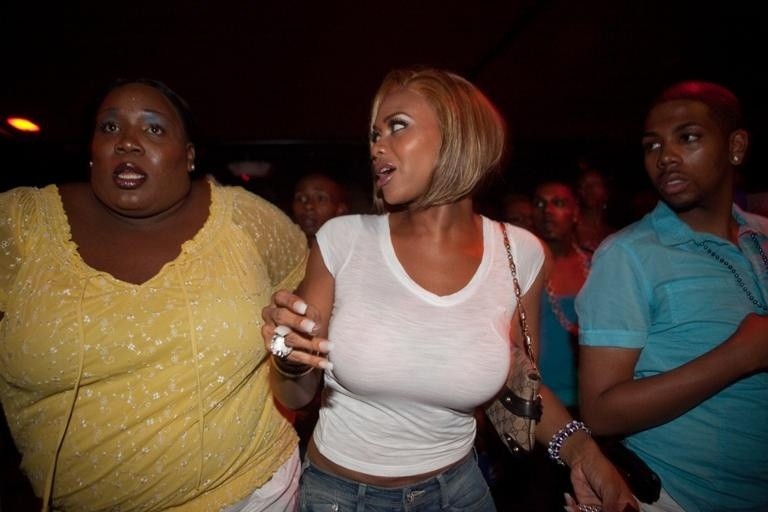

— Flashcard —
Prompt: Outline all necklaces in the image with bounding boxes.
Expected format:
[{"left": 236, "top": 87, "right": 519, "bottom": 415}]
[
  {"left": 703, "top": 236, "right": 767, "bottom": 313},
  {"left": 541, "top": 241, "right": 594, "bottom": 337}
]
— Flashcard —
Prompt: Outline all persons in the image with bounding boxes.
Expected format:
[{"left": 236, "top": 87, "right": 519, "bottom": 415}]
[
  {"left": 1, "top": 81, "right": 310, "bottom": 511},
  {"left": 290, "top": 172, "right": 346, "bottom": 247},
  {"left": 575, "top": 168, "right": 617, "bottom": 245},
  {"left": 572, "top": 80, "right": 767, "bottom": 512},
  {"left": 505, "top": 191, "right": 535, "bottom": 236},
  {"left": 529, "top": 182, "right": 595, "bottom": 408},
  {"left": 262, "top": 68, "right": 638, "bottom": 512}
]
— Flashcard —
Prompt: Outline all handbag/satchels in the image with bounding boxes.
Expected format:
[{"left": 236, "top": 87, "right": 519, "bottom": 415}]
[{"left": 483, "top": 342, "right": 542, "bottom": 461}]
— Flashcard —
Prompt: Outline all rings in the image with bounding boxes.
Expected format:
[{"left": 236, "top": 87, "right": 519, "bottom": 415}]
[{"left": 268, "top": 324, "right": 291, "bottom": 359}]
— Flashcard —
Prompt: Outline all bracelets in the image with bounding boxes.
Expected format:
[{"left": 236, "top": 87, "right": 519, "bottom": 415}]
[
  {"left": 270, "top": 356, "right": 320, "bottom": 378},
  {"left": 546, "top": 421, "right": 590, "bottom": 465}
]
